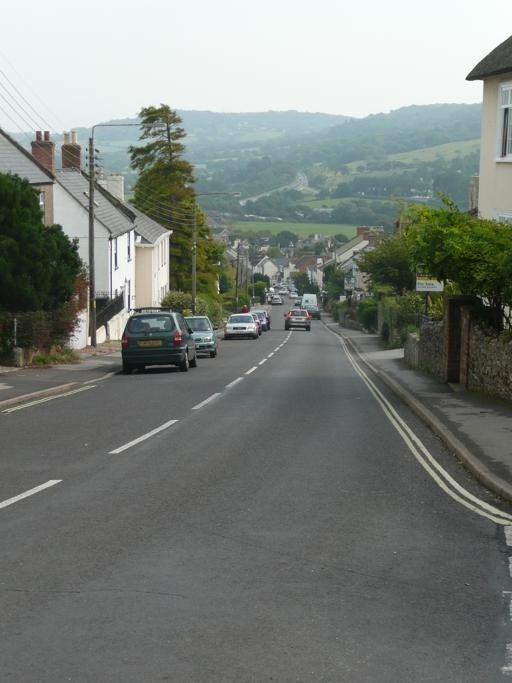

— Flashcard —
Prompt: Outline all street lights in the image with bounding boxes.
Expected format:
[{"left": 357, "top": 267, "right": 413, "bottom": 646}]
[
  {"left": 191, "top": 192, "right": 241, "bottom": 315},
  {"left": 89, "top": 123, "right": 167, "bottom": 349}
]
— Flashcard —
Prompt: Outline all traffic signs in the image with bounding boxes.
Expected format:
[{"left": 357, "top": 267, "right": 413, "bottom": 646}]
[{"left": 415, "top": 264, "right": 444, "bottom": 292}]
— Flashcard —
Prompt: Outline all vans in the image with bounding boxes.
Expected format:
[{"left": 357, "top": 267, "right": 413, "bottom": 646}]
[{"left": 121, "top": 312, "right": 217, "bottom": 373}]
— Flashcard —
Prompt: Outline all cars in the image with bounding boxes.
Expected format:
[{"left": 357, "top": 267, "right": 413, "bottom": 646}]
[{"left": 223, "top": 282, "right": 321, "bottom": 340}]
